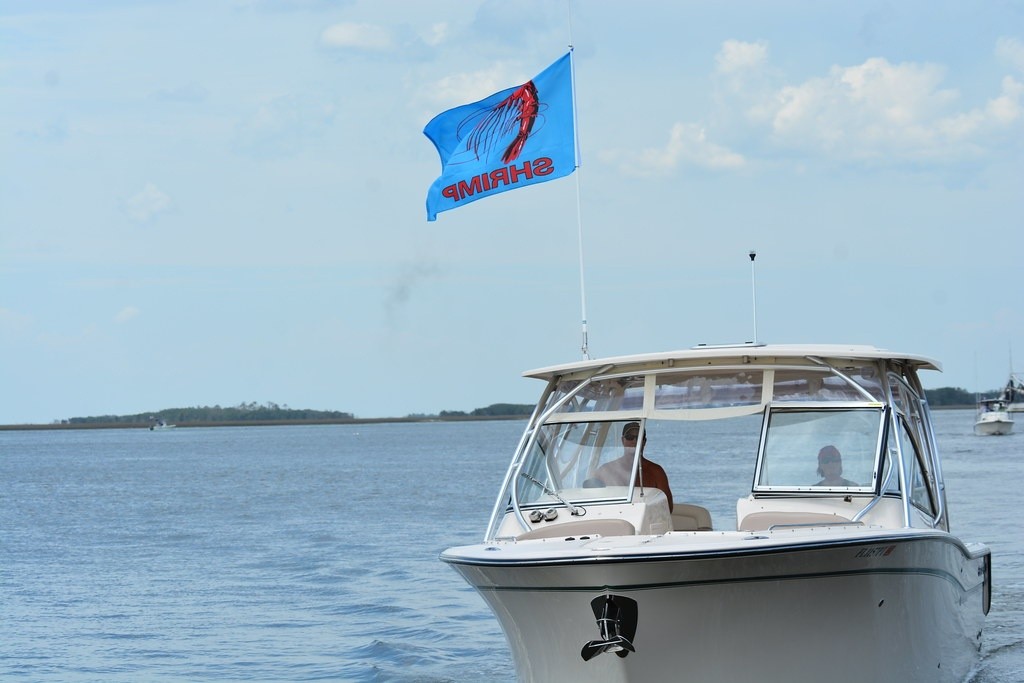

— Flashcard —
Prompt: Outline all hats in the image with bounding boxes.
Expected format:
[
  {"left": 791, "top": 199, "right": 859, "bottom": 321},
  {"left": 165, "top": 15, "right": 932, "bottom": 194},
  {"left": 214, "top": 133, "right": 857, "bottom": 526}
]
[
  {"left": 818, "top": 445, "right": 841, "bottom": 462},
  {"left": 623, "top": 422, "right": 646, "bottom": 438}
]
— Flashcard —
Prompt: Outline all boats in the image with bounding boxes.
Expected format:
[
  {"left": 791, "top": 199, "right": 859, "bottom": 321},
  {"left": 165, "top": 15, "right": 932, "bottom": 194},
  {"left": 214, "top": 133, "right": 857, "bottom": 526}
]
[
  {"left": 149, "top": 417, "right": 176, "bottom": 431},
  {"left": 973, "top": 398, "right": 1010, "bottom": 436},
  {"left": 422, "top": 46, "right": 993, "bottom": 682},
  {"left": 994, "top": 349, "right": 1024, "bottom": 412}
]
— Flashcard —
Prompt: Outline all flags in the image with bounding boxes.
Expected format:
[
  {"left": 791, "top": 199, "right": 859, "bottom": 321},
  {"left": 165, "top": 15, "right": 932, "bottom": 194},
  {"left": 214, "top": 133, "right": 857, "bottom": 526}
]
[{"left": 422, "top": 52, "right": 575, "bottom": 223}]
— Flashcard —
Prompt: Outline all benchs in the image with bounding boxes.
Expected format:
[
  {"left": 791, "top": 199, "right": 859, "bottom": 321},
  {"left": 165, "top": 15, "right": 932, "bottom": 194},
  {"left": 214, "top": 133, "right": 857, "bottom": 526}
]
[{"left": 672, "top": 505, "right": 712, "bottom": 531}]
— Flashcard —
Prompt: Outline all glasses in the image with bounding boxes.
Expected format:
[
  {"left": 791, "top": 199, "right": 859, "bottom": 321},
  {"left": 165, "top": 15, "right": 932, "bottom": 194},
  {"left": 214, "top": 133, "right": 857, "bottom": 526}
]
[
  {"left": 622, "top": 434, "right": 638, "bottom": 441},
  {"left": 820, "top": 456, "right": 841, "bottom": 464}
]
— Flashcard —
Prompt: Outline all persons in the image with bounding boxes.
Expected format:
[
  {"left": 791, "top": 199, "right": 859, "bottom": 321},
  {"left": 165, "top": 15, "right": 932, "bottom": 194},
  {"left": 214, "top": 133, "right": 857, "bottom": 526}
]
[
  {"left": 595, "top": 422, "right": 674, "bottom": 514},
  {"left": 813, "top": 445, "right": 859, "bottom": 486}
]
